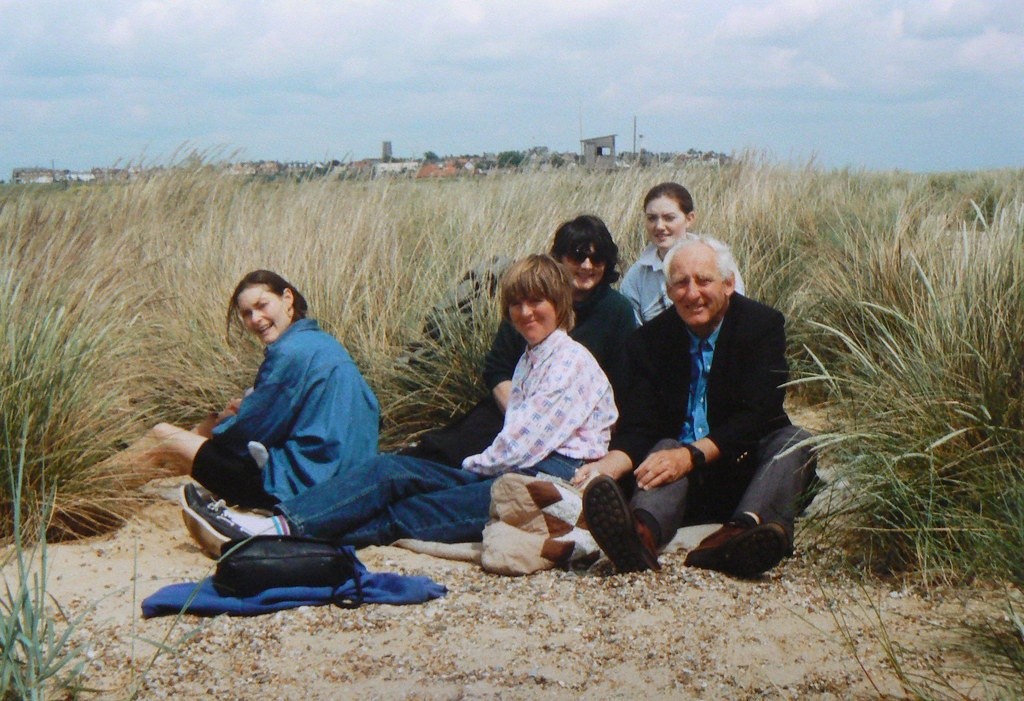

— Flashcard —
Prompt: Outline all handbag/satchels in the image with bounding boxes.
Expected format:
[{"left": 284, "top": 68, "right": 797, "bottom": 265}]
[{"left": 214, "top": 535, "right": 365, "bottom": 609}]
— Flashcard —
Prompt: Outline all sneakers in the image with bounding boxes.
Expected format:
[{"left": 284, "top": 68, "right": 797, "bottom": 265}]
[{"left": 181, "top": 482, "right": 257, "bottom": 556}]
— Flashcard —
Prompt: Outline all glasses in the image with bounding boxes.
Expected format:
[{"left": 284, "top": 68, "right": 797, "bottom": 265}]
[{"left": 565, "top": 246, "right": 606, "bottom": 263}]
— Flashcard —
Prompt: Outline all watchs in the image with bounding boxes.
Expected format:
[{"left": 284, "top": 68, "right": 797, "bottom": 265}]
[{"left": 682, "top": 443, "right": 706, "bottom": 471}]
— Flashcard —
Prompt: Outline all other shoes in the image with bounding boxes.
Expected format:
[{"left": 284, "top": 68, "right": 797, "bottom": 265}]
[
  {"left": 582, "top": 473, "right": 661, "bottom": 572},
  {"left": 684, "top": 520, "right": 793, "bottom": 580}
]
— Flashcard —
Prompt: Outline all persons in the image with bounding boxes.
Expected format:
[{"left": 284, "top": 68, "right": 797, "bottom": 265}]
[
  {"left": 620, "top": 181, "right": 748, "bottom": 328},
  {"left": 394, "top": 214, "right": 638, "bottom": 470},
  {"left": 569, "top": 235, "right": 820, "bottom": 576},
  {"left": 85, "top": 269, "right": 380, "bottom": 510},
  {"left": 177, "top": 252, "right": 619, "bottom": 563}
]
